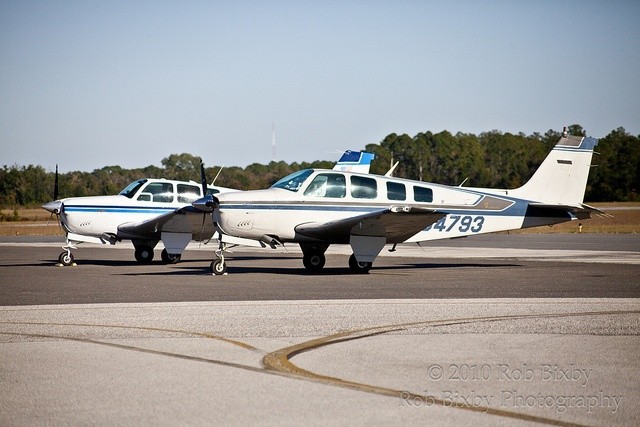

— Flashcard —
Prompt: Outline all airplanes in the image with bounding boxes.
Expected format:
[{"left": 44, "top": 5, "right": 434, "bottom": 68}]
[
  {"left": 192, "top": 127, "right": 614, "bottom": 273},
  {"left": 41, "top": 162, "right": 334, "bottom": 266}
]
